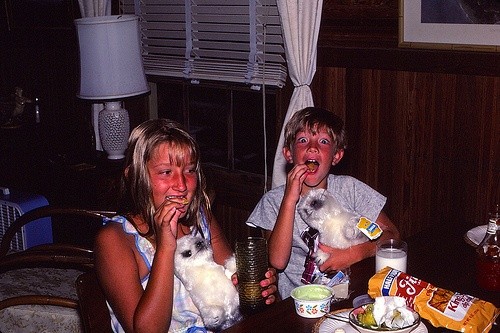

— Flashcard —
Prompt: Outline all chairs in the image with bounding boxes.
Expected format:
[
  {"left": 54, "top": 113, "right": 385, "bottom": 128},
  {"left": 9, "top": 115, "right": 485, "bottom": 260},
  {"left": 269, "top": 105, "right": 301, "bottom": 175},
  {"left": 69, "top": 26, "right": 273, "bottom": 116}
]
[{"left": 0, "top": 168, "right": 217, "bottom": 332}]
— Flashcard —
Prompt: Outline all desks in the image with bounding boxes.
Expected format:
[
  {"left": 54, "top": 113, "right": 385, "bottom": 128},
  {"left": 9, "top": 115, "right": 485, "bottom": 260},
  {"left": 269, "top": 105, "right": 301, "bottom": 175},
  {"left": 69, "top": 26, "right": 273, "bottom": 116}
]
[
  {"left": 44, "top": 152, "right": 128, "bottom": 250},
  {"left": 221, "top": 222, "right": 500, "bottom": 333}
]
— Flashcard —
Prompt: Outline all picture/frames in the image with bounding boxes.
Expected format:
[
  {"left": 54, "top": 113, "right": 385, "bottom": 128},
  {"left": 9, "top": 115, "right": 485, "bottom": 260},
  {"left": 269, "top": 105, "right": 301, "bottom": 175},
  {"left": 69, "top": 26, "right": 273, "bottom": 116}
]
[{"left": 398, "top": 0, "right": 500, "bottom": 53}]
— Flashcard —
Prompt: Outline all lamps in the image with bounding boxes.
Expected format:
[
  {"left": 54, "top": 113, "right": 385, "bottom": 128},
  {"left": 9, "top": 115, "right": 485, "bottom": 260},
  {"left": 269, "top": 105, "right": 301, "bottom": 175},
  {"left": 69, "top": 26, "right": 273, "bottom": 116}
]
[{"left": 77, "top": 14, "right": 151, "bottom": 162}]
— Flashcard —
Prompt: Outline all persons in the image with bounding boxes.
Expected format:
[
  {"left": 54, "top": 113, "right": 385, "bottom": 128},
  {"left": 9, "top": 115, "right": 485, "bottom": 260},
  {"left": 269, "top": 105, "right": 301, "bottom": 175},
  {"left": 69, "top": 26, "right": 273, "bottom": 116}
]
[
  {"left": 246, "top": 107, "right": 400, "bottom": 300},
  {"left": 92, "top": 117, "right": 277, "bottom": 333}
]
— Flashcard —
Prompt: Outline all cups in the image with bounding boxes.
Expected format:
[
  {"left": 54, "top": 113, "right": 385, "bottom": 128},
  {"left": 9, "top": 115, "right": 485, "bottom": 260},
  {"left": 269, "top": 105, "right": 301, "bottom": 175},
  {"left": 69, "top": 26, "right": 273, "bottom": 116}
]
[
  {"left": 236, "top": 237, "right": 269, "bottom": 314},
  {"left": 375, "top": 238, "right": 407, "bottom": 273}
]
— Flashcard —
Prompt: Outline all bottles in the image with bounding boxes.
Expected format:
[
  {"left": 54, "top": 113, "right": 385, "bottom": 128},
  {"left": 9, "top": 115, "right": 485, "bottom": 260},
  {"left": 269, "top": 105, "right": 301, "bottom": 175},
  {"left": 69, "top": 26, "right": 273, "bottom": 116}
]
[{"left": 474, "top": 202, "right": 500, "bottom": 295}]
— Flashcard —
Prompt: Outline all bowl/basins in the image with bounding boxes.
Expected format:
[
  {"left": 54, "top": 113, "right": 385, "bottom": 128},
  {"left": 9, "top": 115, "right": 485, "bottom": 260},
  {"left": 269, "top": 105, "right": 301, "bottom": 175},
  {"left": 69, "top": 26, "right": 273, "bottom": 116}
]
[
  {"left": 351, "top": 303, "right": 421, "bottom": 333},
  {"left": 290, "top": 284, "right": 334, "bottom": 319}
]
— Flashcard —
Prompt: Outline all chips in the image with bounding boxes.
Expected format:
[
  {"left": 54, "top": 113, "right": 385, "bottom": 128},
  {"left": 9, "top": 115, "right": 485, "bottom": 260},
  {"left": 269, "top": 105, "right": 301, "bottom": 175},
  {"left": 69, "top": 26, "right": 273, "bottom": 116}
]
[{"left": 429, "top": 287, "right": 454, "bottom": 313}]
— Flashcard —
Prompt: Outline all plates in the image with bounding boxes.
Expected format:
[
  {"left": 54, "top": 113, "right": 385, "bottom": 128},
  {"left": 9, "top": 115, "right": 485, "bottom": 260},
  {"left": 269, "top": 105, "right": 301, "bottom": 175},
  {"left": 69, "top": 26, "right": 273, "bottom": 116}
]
[
  {"left": 467, "top": 224, "right": 500, "bottom": 245},
  {"left": 318, "top": 311, "right": 428, "bottom": 333}
]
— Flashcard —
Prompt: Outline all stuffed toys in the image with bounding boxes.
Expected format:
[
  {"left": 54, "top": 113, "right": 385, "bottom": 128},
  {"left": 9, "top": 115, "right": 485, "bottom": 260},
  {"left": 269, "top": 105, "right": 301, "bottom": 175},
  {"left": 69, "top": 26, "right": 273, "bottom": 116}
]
[
  {"left": 173, "top": 234, "right": 244, "bottom": 330},
  {"left": 298, "top": 188, "right": 369, "bottom": 265}
]
